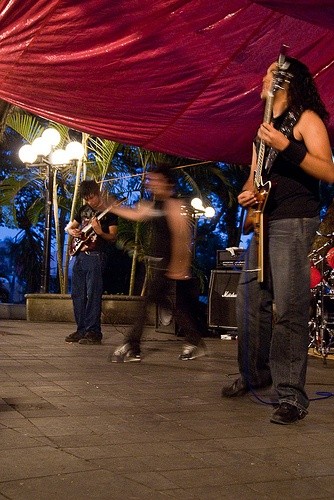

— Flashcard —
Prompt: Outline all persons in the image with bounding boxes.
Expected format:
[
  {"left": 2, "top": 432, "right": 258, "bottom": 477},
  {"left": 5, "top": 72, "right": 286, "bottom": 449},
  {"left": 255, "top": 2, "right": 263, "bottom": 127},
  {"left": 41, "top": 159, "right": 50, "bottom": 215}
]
[
  {"left": 101, "top": 170, "right": 208, "bottom": 362},
  {"left": 220, "top": 57, "right": 334, "bottom": 425},
  {"left": 65, "top": 180, "right": 119, "bottom": 345}
]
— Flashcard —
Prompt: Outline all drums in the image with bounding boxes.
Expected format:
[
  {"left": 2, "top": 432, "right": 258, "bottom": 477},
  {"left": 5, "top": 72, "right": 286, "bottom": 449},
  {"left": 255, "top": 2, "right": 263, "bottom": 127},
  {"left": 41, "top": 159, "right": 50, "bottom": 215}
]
[{"left": 307, "top": 293, "right": 334, "bottom": 350}]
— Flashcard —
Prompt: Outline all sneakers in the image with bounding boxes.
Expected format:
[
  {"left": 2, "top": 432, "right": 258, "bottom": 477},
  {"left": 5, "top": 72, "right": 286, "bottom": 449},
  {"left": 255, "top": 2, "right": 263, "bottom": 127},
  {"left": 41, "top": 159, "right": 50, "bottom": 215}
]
[
  {"left": 177, "top": 342, "right": 208, "bottom": 360},
  {"left": 79, "top": 331, "right": 102, "bottom": 345},
  {"left": 64, "top": 332, "right": 83, "bottom": 342},
  {"left": 269, "top": 402, "right": 307, "bottom": 424},
  {"left": 111, "top": 342, "right": 142, "bottom": 363},
  {"left": 223, "top": 373, "right": 273, "bottom": 404}
]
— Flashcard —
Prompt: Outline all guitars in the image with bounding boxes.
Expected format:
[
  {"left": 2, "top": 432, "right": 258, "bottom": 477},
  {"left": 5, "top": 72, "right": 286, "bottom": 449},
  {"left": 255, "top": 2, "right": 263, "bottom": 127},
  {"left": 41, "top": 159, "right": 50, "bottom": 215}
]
[
  {"left": 67, "top": 195, "right": 128, "bottom": 257},
  {"left": 241, "top": 43, "right": 294, "bottom": 245}
]
